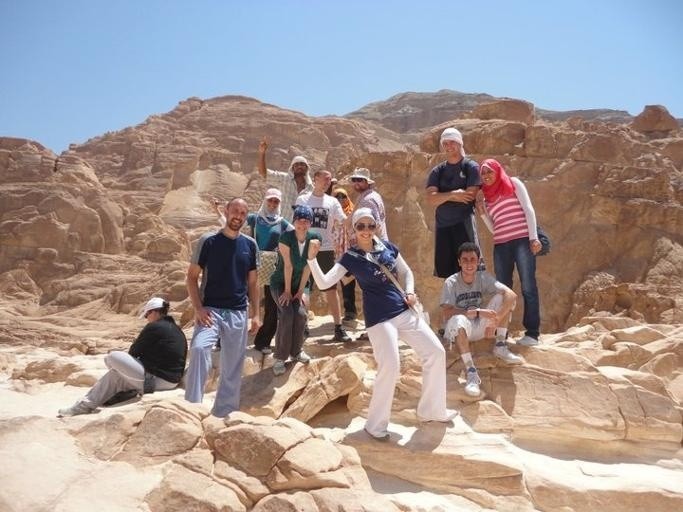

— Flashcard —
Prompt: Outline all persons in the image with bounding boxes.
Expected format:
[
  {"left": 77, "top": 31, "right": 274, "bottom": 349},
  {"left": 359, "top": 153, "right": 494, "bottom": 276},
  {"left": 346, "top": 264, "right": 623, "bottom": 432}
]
[
  {"left": 58, "top": 297, "right": 187, "bottom": 414},
  {"left": 211, "top": 188, "right": 295, "bottom": 355},
  {"left": 270, "top": 205, "right": 321, "bottom": 376},
  {"left": 475, "top": 158, "right": 542, "bottom": 345},
  {"left": 258, "top": 135, "right": 312, "bottom": 224},
  {"left": 295, "top": 170, "right": 352, "bottom": 342},
  {"left": 326, "top": 178, "right": 356, "bottom": 322},
  {"left": 306, "top": 207, "right": 458, "bottom": 440},
  {"left": 339, "top": 167, "right": 389, "bottom": 320},
  {"left": 439, "top": 243, "right": 522, "bottom": 396},
  {"left": 184, "top": 197, "right": 261, "bottom": 419},
  {"left": 425, "top": 127, "right": 486, "bottom": 278}
]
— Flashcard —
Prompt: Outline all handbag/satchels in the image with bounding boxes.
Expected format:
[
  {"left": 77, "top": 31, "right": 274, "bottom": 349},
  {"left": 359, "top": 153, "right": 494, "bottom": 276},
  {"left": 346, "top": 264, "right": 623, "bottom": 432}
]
[
  {"left": 346, "top": 250, "right": 420, "bottom": 318},
  {"left": 252, "top": 220, "right": 275, "bottom": 286},
  {"left": 534, "top": 227, "right": 550, "bottom": 257}
]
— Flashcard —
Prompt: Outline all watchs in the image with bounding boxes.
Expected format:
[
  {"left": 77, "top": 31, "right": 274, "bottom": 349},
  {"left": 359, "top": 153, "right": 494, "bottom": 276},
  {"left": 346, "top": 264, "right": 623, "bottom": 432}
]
[{"left": 476, "top": 308, "right": 480, "bottom": 318}]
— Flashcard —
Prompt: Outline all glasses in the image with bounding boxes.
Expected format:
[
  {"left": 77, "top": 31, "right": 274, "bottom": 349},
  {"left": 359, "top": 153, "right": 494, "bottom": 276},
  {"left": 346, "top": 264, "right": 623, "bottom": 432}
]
[{"left": 356, "top": 222, "right": 375, "bottom": 230}]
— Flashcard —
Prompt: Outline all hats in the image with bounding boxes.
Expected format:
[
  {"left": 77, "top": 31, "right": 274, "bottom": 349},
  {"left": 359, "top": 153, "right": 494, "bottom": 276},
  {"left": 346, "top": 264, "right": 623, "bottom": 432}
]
[
  {"left": 352, "top": 207, "right": 376, "bottom": 229},
  {"left": 350, "top": 167, "right": 375, "bottom": 184},
  {"left": 138, "top": 297, "right": 169, "bottom": 319},
  {"left": 264, "top": 188, "right": 281, "bottom": 201},
  {"left": 292, "top": 205, "right": 313, "bottom": 221}
]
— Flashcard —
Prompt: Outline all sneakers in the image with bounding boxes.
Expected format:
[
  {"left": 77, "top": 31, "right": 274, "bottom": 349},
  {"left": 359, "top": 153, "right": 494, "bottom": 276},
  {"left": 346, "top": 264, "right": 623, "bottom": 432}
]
[
  {"left": 335, "top": 329, "right": 351, "bottom": 341},
  {"left": 465, "top": 371, "right": 481, "bottom": 396},
  {"left": 418, "top": 409, "right": 458, "bottom": 422},
  {"left": 516, "top": 335, "right": 538, "bottom": 345},
  {"left": 363, "top": 421, "right": 391, "bottom": 440},
  {"left": 343, "top": 316, "right": 352, "bottom": 324},
  {"left": 290, "top": 349, "right": 309, "bottom": 363},
  {"left": 493, "top": 345, "right": 522, "bottom": 364},
  {"left": 273, "top": 357, "right": 286, "bottom": 375},
  {"left": 58, "top": 401, "right": 91, "bottom": 416},
  {"left": 261, "top": 346, "right": 271, "bottom": 354}
]
